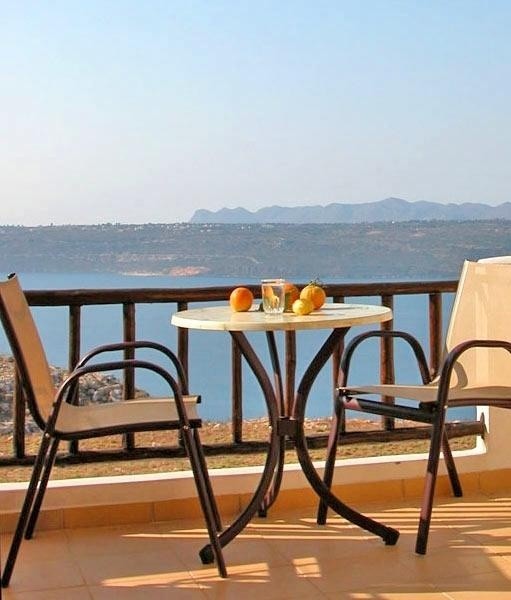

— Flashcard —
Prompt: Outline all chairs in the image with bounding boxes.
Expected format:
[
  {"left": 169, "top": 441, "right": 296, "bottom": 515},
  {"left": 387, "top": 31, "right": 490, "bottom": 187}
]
[
  {"left": 316, "top": 259, "right": 511, "bottom": 555},
  {"left": 0, "top": 272, "right": 228, "bottom": 588}
]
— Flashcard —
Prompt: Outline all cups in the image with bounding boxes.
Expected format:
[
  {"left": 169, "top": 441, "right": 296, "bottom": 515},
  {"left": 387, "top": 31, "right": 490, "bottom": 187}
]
[{"left": 260, "top": 279, "right": 286, "bottom": 315}]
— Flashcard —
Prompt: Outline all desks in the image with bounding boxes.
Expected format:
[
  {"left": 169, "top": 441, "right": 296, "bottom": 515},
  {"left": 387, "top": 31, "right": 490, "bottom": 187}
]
[{"left": 170, "top": 303, "right": 400, "bottom": 565}]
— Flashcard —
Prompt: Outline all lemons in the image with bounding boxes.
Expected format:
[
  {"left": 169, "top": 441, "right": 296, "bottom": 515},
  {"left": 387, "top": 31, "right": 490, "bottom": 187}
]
[{"left": 292, "top": 299, "right": 314, "bottom": 314}]
